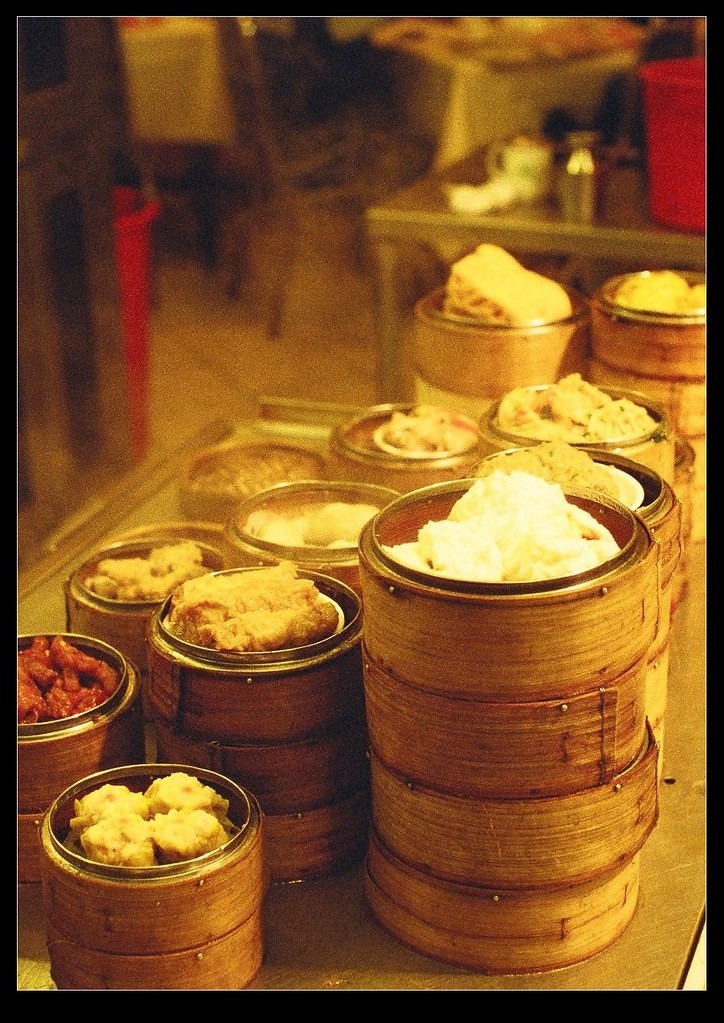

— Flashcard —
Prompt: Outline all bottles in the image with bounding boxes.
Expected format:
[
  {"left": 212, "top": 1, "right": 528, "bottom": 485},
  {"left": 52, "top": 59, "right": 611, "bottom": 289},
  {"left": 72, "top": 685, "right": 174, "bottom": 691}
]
[{"left": 545, "top": 110, "right": 638, "bottom": 226}]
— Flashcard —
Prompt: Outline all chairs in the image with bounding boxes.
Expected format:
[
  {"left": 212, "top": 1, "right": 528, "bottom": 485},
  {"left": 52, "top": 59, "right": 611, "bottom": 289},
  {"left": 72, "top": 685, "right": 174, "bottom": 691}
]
[{"left": 224, "top": 19, "right": 416, "bottom": 342}]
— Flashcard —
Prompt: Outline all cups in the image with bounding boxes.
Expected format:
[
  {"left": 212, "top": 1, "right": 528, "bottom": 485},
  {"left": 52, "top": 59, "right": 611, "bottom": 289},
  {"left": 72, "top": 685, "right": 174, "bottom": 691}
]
[{"left": 485, "top": 132, "right": 553, "bottom": 204}]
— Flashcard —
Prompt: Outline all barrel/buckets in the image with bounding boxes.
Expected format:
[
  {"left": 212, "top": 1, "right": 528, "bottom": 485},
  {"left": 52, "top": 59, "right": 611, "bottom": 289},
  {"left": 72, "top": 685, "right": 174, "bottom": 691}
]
[
  {"left": 638, "top": 59, "right": 706, "bottom": 233},
  {"left": 112, "top": 187, "right": 162, "bottom": 459}
]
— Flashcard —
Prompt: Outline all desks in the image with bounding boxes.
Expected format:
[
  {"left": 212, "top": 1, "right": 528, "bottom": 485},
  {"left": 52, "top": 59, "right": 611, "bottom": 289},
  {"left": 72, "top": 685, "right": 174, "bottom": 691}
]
[
  {"left": 16, "top": 402, "right": 710, "bottom": 992},
  {"left": 125, "top": 13, "right": 707, "bottom": 408}
]
